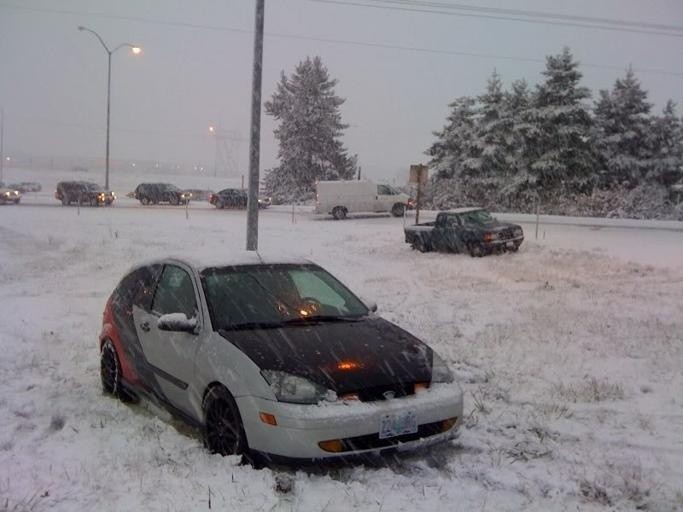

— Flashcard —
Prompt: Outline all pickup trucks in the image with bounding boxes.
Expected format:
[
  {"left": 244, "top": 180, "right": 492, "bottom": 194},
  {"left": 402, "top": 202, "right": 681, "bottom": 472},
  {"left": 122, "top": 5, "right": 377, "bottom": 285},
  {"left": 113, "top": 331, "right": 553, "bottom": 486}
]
[{"left": 404, "top": 207, "right": 523, "bottom": 256}]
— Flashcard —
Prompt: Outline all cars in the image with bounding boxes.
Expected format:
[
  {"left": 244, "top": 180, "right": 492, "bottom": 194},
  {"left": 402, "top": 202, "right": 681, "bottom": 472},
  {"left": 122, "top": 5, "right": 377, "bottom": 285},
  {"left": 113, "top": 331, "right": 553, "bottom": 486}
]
[
  {"left": 0, "top": 184, "right": 21, "bottom": 204},
  {"left": 210, "top": 189, "right": 272, "bottom": 210},
  {"left": 99, "top": 250, "right": 464, "bottom": 469}
]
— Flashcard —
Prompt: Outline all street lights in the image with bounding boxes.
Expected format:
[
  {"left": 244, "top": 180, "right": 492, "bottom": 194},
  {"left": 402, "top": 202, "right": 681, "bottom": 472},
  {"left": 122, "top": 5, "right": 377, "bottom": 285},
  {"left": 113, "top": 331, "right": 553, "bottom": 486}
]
[{"left": 78, "top": 26, "right": 141, "bottom": 189}]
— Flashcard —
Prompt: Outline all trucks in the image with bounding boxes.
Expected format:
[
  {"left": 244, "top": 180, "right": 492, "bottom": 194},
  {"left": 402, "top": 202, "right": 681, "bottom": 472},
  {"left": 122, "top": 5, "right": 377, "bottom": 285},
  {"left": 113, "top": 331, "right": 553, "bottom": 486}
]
[{"left": 316, "top": 180, "right": 417, "bottom": 219}]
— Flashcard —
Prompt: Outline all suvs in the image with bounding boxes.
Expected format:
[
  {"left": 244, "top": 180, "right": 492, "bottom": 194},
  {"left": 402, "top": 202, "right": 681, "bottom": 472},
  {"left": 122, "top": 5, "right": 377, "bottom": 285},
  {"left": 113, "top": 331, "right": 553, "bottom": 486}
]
[
  {"left": 135, "top": 183, "right": 191, "bottom": 206},
  {"left": 10, "top": 183, "right": 41, "bottom": 192},
  {"left": 56, "top": 181, "right": 114, "bottom": 207}
]
